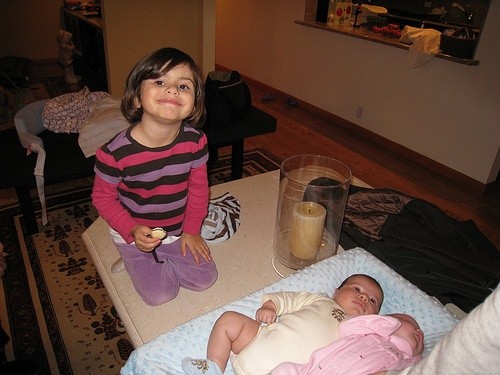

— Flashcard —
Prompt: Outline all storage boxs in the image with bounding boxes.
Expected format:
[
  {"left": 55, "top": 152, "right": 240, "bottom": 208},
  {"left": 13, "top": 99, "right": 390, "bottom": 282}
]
[{"left": 439, "top": 34, "right": 479, "bottom": 60}]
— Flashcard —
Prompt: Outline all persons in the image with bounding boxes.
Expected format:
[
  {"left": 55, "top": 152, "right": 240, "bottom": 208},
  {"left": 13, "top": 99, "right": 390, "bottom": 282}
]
[
  {"left": 264, "top": 312, "right": 424, "bottom": 375},
  {"left": 91, "top": 47, "right": 219, "bottom": 307},
  {"left": 181, "top": 273, "right": 385, "bottom": 375}
]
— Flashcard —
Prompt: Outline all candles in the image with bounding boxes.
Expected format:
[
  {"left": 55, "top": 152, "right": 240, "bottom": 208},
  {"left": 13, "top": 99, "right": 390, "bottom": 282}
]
[{"left": 289, "top": 201, "right": 327, "bottom": 260}]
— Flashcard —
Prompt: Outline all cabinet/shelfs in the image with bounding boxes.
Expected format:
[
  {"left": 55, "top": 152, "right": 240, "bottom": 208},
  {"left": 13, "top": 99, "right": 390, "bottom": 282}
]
[{"left": 63, "top": 8, "right": 108, "bottom": 94}]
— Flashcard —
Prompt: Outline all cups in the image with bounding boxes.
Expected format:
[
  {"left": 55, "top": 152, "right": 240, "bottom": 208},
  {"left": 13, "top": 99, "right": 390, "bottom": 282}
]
[{"left": 272, "top": 154, "right": 351, "bottom": 278}]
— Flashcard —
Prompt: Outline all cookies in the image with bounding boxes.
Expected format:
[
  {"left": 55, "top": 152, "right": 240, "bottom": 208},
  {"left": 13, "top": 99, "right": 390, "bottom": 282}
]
[{"left": 151, "top": 228, "right": 166, "bottom": 240}]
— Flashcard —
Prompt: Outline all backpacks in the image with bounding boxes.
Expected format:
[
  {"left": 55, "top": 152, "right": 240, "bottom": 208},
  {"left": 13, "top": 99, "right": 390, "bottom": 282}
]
[{"left": 205, "top": 70, "right": 246, "bottom": 123}]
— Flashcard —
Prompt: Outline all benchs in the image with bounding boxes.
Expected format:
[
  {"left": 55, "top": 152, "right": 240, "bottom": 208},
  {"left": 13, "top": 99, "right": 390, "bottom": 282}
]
[{"left": 0, "top": 105, "right": 277, "bottom": 235}]
